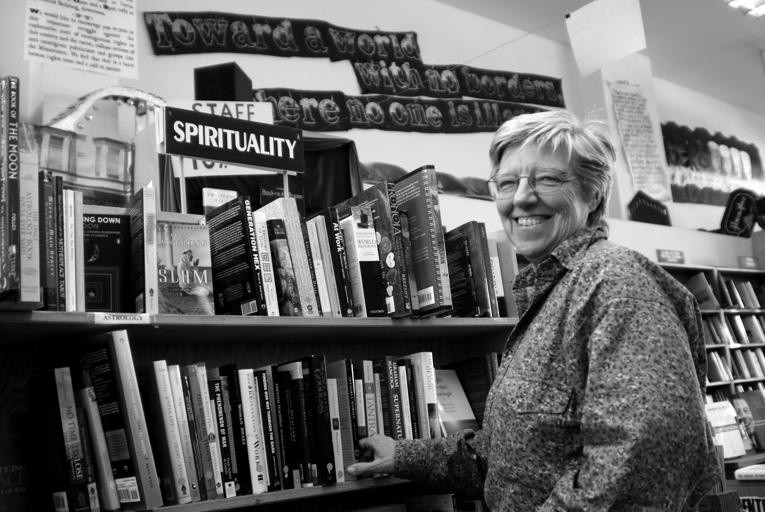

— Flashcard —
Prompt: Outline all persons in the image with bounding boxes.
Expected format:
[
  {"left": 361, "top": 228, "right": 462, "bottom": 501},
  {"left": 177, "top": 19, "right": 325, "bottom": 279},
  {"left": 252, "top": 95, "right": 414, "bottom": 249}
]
[
  {"left": 683, "top": 272, "right": 765, "bottom": 481},
  {"left": 345, "top": 109, "right": 748, "bottom": 512}
]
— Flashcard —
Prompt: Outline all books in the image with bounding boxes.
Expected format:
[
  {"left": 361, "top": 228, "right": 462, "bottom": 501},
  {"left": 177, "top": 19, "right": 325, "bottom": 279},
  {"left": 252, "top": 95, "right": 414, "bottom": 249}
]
[{"left": 0, "top": 76, "right": 525, "bottom": 512}]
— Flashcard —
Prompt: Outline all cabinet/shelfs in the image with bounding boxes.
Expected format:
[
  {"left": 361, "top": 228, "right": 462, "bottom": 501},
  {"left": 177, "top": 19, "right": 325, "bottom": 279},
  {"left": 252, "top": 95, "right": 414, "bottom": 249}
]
[
  {"left": 0, "top": 311, "right": 522, "bottom": 512},
  {"left": 654, "top": 264, "right": 764, "bottom": 511}
]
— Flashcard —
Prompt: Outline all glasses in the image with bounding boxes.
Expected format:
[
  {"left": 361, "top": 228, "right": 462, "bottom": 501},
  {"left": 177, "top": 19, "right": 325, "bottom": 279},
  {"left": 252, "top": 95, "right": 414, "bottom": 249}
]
[{"left": 486, "top": 168, "right": 575, "bottom": 198}]
